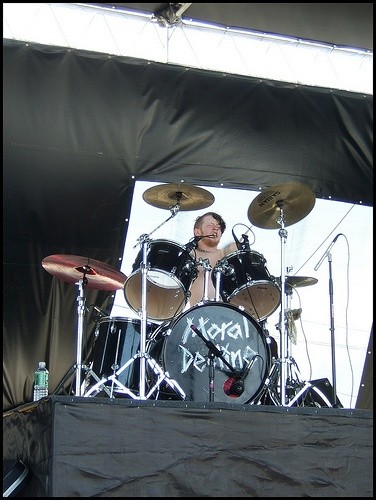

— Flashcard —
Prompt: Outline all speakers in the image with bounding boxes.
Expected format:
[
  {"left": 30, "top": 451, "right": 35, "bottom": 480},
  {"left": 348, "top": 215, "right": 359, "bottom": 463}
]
[{"left": 294, "top": 378, "right": 345, "bottom": 409}]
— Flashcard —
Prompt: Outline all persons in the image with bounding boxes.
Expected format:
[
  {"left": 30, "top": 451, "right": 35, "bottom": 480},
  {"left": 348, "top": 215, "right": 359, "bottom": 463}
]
[{"left": 186, "top": 212, "right": 238, "bottom": 308}]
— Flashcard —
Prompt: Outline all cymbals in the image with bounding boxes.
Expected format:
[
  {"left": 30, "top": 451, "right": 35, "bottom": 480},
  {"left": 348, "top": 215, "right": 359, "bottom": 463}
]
[
  {"left": 142, "top": 182, "right": 216, "bottom": 212},
  {"left": 247, "top": 181, "right": 316, "bottom": 230},
  {"left": 275, "top": 275, "right": 319, "bottom": 289},
  {"left": 40, "top": 253, "right": 128, "bottom": 291}
]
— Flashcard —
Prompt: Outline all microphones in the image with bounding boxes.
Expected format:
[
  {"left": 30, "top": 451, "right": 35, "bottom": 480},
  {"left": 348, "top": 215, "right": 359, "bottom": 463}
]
[
  {"left": 230, "top": 382, "right": 244, "bottom": 395},
  {"left": 233, "top": 233, "right": 242, "bottom": 249},
  {"left": 314, "top": 236, "right": 338, "bottom": 271}
]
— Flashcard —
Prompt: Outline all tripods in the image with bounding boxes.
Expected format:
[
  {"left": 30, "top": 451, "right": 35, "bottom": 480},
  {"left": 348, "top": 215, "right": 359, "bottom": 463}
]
[
  {"left": 256, "top": 204, "right": 331, "bottom": 409},
  {"left": 51, "top": 205, "right": 191, "bottom": 402}
]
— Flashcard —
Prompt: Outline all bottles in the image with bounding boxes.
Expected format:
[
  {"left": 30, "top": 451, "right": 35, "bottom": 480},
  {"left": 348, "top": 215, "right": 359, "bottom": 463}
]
[{"left": 33, "top": 362, "right": 49, "bottom": 401}]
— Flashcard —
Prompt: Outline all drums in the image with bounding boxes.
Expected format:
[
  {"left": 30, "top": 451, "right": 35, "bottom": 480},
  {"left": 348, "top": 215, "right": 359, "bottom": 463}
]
[
  {"left": 123, "top": 237, "right": 199, "bottom": 322},
  {"left": 145, "top": 301, "right": 273, "bottom": 405},
  {"left": 211, "top": 249, "right": 281, "bottom": 323},
  {"left": 82, "top": 315, "right": 163, "bottom": 398}
]
